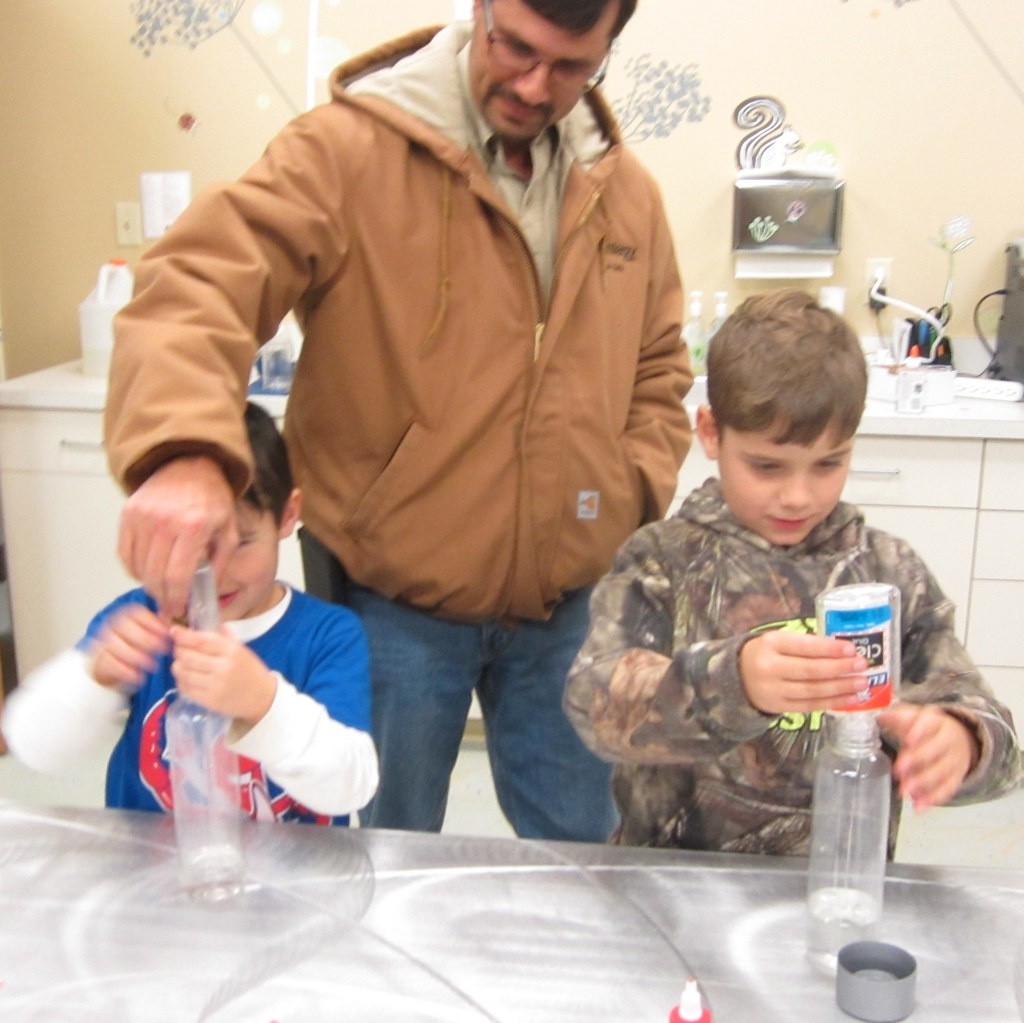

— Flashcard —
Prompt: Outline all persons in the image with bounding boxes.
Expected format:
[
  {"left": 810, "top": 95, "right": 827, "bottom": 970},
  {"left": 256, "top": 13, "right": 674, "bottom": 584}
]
[
  {"left": 557, "top": 286, "right": 1024, "bottom": 863},
  {"left": 0, "top": 397, "right": 381, "bottom": 826},
  {"left": 102, "top": 0, "right": 697, "bottom": 842}
]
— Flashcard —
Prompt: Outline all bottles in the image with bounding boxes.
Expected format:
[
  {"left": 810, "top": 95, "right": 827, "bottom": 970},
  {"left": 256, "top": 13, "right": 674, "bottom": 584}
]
[
  {"left": 78, "top": 258, "right": 136, "bottom": 376},
  {"left": 813, "top": 582, "right": 902, "bottom": 734},
  {"left": 166, "top": 694, "right": 246, "bottom": 909},
  {"left": 262, "top": 322, "right": 293, "bottom": 388},
  {"left": 171, "top": 561, "right": 219, "bottom": 631},
  {"left": 807, "top": 713, "right": 893, "bottom": 982}
]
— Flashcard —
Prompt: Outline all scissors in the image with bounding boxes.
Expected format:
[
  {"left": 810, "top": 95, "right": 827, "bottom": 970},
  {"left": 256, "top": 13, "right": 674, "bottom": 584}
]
[{"left": 926, "top": 303, "right": 952, "bottom": 328}]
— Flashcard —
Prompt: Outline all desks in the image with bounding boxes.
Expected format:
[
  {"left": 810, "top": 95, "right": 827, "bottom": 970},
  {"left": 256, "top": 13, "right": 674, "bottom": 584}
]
[{"left": 0, "top": 800, "right": 1024, "bottom": 1023}]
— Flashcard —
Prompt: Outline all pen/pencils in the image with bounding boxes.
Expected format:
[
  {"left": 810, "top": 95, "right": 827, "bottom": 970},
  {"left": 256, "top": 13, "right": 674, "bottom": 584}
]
[{"left": 903, "top": 318, "right": 952, "bottom": 367}]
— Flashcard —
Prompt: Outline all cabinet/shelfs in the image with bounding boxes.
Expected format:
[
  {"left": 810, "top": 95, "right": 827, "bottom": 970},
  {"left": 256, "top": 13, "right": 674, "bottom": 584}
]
[{"left": 0, "top": 355, "right": 1024, "bottom": 863}]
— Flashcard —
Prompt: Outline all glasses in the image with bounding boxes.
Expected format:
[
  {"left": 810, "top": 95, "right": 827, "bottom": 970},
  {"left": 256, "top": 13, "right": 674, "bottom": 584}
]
[{"left": 483, "top": 1, "right": 611, "bottom": 96}]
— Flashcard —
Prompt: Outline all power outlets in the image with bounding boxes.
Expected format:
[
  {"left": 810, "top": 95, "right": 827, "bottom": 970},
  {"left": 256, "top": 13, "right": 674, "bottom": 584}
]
[{"left": 866, "top": 258, "right": 892, "bottom": 302}]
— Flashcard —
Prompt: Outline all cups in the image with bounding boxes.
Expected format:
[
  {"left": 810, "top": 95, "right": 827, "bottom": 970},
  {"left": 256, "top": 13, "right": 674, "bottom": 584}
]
[{"left": 837, "top": 941, "right": 917, "bottom": 1022}]
